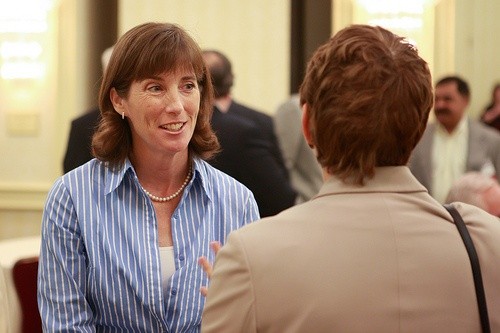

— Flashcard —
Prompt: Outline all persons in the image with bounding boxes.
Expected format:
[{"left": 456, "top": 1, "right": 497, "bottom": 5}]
[
  {"left": 37, "top": 22, "right": 261, "bottom": 333},
  {"left": 201, "top": 24, "right": 500, "bottom": 333},
  {"left": 60, "top": 44, "right": 500, "bottom": 219}
]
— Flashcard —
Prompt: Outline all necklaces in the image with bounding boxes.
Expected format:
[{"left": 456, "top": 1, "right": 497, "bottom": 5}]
[{"left": 134, "top": 159, "right": 192, "bottom": 202}]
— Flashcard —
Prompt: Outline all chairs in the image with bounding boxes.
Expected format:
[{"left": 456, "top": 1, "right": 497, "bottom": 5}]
[{"left": 12, "top": 256, "right": 42, "bottom": 333}]
